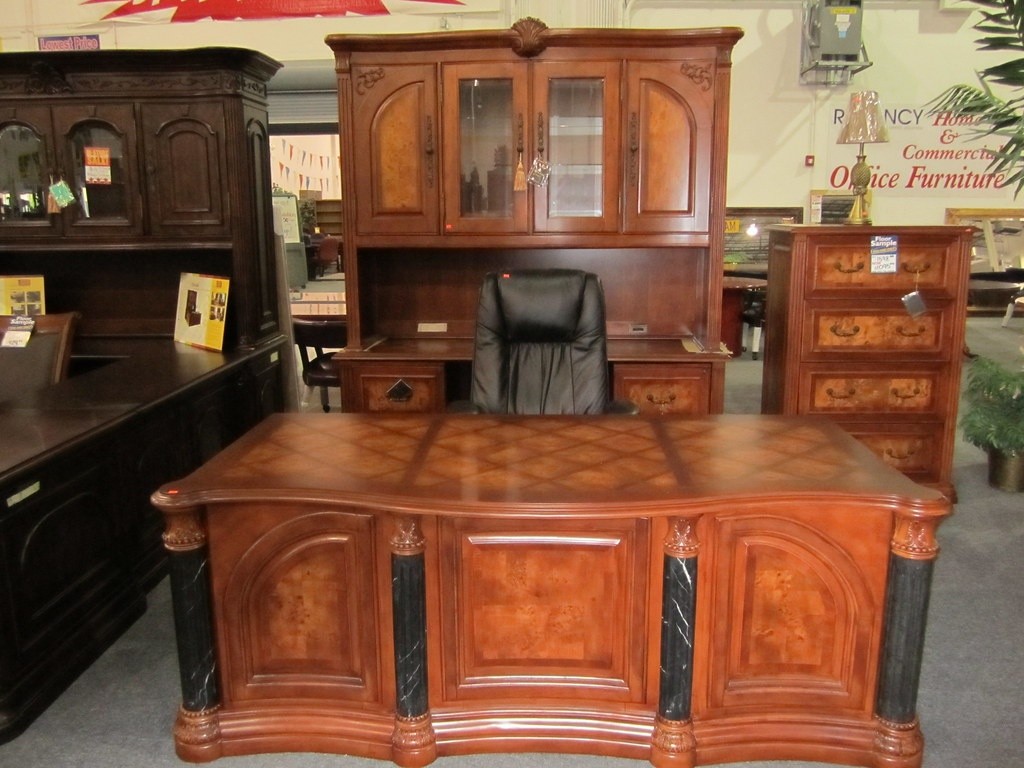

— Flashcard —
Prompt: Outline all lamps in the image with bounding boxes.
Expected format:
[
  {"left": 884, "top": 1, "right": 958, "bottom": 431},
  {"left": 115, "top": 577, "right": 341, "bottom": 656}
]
[{"left": 836, "top": 91, "right": 890, "bottom": 225}]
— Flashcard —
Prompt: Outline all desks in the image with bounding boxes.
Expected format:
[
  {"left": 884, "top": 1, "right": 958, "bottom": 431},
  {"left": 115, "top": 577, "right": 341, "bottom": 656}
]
[
  {"left": 721, "top": 276, "right": 767, "bottom": 358},
  {"left": 148, "top": 405, "right": 954, "bottom": 768}
]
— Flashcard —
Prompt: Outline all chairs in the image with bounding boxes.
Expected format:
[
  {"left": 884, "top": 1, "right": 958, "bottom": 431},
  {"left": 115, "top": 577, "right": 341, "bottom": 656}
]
[
  {"left": 444, "top": 268, "right": 639, "bottom": 413},
  {"left": 290, "top": 315, "right": 347, "bottom": 413},
  {"left": 0, "top": 311, "right": 80, "bottom": 400}
]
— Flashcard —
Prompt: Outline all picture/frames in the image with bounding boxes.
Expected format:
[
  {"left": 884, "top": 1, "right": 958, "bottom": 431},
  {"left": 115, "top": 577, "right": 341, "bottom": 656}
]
[
  {"left": 810, "top": 188, "right": 872, "bottom": 221},
  {"left": 945, "top": 207, "right": 1023, "bottom": 273},
  {"left": 723, "top": 206, "right": 803, "bottom": 263}
]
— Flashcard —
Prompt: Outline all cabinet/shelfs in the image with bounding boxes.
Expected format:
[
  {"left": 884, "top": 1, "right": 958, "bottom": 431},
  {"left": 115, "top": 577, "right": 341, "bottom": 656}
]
[
  {"left": 0, "top": 46, "right": 287, "bottom": 348},
  {"left": 326, "top": 16, "right": 745, "bottom": 417},
  {"left": 759, "top": 223, "right": 978, "bottom": 503},
  {"left": 0, "top": 100, "right": 143, "bottom": 244}
]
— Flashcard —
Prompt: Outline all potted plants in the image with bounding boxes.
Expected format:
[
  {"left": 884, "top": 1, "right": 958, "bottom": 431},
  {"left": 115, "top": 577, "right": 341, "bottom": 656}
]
[{"left": 958, "top": 355, "right": 1024, "bottom": 493}]
